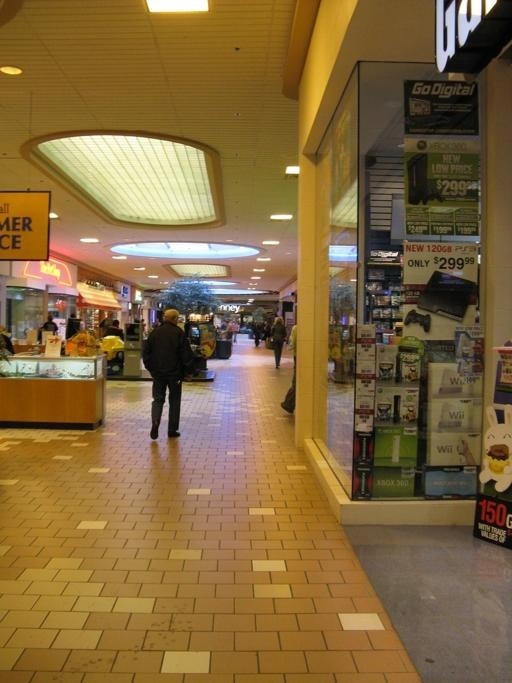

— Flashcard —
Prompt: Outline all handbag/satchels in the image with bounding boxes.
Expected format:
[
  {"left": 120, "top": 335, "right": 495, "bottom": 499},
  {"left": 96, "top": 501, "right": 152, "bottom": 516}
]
[{"left": 265, "top": 337, "right": 277, "bottom": 349}]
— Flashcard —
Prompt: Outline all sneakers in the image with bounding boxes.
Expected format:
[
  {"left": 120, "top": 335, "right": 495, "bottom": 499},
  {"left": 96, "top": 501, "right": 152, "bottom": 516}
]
[
  {"left": 150, "top": 421, "right": 160, "bottom": 440},
  {"left": 167, "top": 431, "right": 181, "bottom": 437}
]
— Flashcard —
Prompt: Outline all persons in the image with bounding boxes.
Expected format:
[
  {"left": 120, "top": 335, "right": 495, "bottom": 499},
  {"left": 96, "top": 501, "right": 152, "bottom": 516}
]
[
  {"left": 281, "top": 323, "right": 297, "bottom": 413},
  {"left": 270, "top": 316, "right": 287, "bottom": 368},
  {"left": 183, "top": 318, "right": 192, "bottom": 337},
  {"left": 0, "top": 324, "right": 16, "bottom": 354},
  {"left": 142, "top": 307, "right": 195, "bottom": 439},
  {"left": 78, "top": 317, "right": 85, "bottom": 330},
  {"left": 220, "top": 314, "right": 272, "bottom": 347},
  {"left": 102, "top": 318, "right": 124, "bottom": 340},
  {"left": 70, "top": 313, "right": 76, "bottom": 318},
  {"left": 44, "top": 314, "right": 58, "bottom": 333}
]
